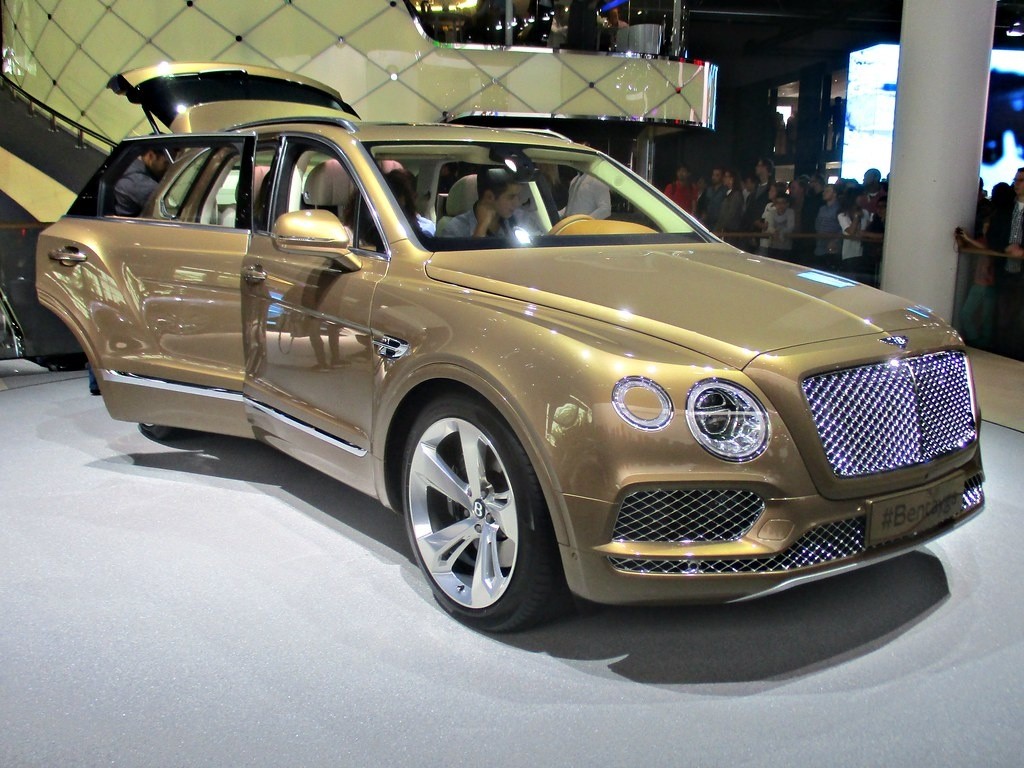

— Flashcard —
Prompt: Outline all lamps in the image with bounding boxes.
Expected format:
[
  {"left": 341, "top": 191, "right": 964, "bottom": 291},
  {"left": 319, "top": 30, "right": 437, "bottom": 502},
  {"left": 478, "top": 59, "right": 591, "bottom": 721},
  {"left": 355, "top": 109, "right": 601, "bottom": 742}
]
[{"left": 1006, "top": 21, "right": 1024, "bottom": 36}]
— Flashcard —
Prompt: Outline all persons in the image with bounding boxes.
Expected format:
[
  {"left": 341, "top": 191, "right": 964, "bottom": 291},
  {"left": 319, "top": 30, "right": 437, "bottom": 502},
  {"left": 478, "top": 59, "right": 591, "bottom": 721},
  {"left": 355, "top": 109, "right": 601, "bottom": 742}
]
[
  {"left": 446, "top": 169, "right": 545, "bottom": 238},
  {"left": 392, "top": 169, "right": 436, "bottom": 236},
  {"left": 663, "top": 157, "right": 1024, "bottom": 363},
  {"left": 311, "top": 320, "right": 344, "bottom": 374},
  {"left": 457, "top": 0, "right": 629, "bottom": 52},
  {"left": 90, "top": 131, "right": 177, "bottom": 394},
  {"left": 558, "top": 141, "right": 611, "bottom": 220}
]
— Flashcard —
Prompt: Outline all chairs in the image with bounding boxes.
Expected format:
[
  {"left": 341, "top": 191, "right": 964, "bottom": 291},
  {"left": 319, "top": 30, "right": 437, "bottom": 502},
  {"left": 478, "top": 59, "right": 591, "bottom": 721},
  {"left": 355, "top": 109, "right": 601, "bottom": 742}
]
[
  {"left": 220, "top": 166, "right": 266, "bottom": 233},
  {"left": 435, "top": 173, "right": 480, "bottom": 242},
  {"left": 303, "top": 159, "right": 364, "bottom": 244}
]
